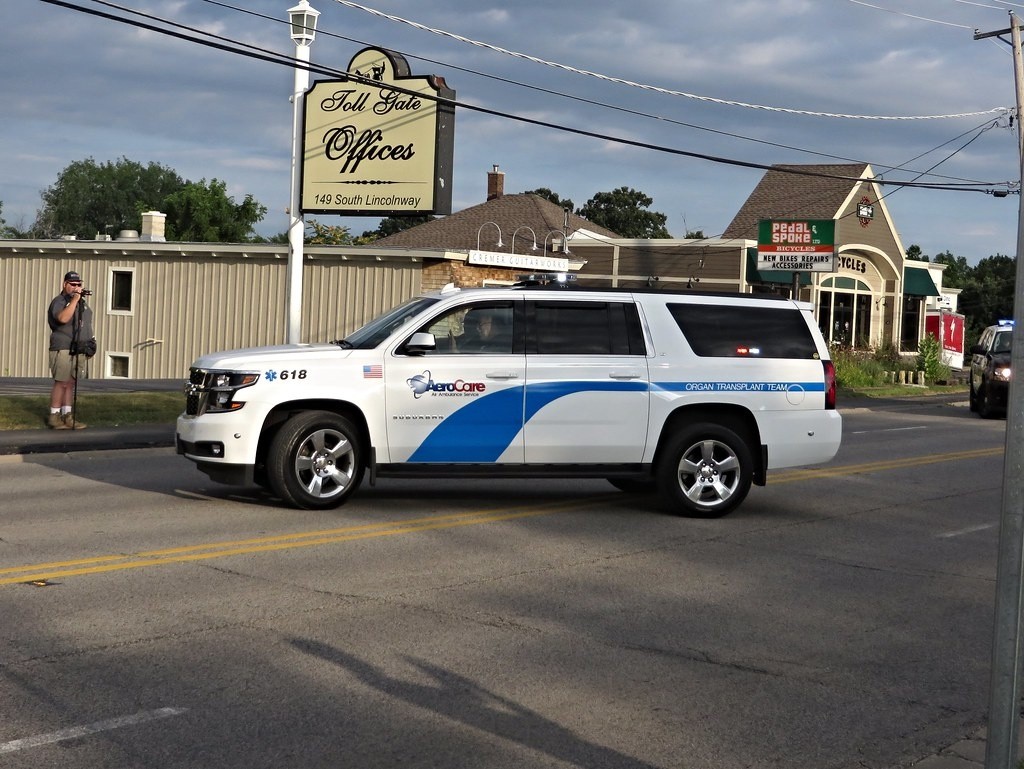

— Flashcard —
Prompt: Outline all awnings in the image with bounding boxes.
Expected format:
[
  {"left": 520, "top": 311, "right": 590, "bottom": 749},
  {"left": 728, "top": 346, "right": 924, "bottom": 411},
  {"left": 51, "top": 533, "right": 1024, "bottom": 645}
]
[
  {"left": 746, "top": 247, "right": 813, "bottom": 285},
  {"left": 904, "top": 266, "right": 940, "bottom": 296}
]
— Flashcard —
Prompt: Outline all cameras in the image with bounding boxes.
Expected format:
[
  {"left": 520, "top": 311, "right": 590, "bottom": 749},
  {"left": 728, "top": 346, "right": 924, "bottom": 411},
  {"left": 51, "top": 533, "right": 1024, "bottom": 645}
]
[{"left": 81, "top": 288, "right": 94, "bottom": 296}]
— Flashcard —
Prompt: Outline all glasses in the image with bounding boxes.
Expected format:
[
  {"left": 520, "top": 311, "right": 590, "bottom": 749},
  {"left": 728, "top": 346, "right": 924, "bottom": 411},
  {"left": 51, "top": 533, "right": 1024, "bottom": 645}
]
[
  {"left": 478, "top": 322, "right": 492, "bottom": 326},
  {"left": 66, "top": 282, "right": 81, "bottom": 287}
]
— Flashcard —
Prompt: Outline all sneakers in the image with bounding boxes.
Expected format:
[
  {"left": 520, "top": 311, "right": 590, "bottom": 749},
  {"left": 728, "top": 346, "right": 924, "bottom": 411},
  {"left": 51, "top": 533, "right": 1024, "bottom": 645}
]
[{"left": 47, "top": 412, "right": 87, "bottom": 430}]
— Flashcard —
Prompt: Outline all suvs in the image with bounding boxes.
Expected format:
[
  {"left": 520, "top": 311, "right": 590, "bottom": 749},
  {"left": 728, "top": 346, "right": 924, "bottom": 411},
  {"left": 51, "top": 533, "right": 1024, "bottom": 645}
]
[
  {"left": 967, "top": 321, "right": 1015, "bottom": 416},
  {"left": 175, "top": 281, "right": 844, "bottom": 517}
]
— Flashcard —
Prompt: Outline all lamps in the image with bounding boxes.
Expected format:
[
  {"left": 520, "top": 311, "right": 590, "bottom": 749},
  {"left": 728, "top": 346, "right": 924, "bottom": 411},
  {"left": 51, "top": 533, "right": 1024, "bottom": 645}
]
[
  {"left": 686, "top": 275, "right": 700, "bottom": 289},
  {"left": 477, "top": 221, "right": 505, "bottom": 251},
  {"left": 544, "top": 230, "right": 572, "bottom": 257},
  {"left": 646, "top": 274, "right": 659, "bottom": 287},
  {"left": 512, "top": 226, "right": 539, "bottom": 254}
]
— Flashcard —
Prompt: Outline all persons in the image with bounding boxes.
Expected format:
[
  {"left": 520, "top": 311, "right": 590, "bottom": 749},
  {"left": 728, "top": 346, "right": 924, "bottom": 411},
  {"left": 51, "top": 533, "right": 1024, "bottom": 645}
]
[
  {"left": 1000, "top": 334, "right": 1010, "bottom": 347},
  {"left": 448, "top": 309, "right": 510, "bottom": 352},
  {"left": 46, "top": 271, "right": 94, "bottom": 429}
]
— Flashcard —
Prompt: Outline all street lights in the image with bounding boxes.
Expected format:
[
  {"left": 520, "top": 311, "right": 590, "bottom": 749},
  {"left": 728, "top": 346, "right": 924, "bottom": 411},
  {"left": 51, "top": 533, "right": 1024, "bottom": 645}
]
[{"left": 287, "top": 1, "right": 323, "bottom": 344}]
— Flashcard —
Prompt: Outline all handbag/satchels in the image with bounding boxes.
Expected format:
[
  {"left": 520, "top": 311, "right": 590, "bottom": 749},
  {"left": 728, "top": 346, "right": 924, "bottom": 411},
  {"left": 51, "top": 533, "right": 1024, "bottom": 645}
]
[{"left": 69, "top": 337, "right": 96, "bottom": 359}]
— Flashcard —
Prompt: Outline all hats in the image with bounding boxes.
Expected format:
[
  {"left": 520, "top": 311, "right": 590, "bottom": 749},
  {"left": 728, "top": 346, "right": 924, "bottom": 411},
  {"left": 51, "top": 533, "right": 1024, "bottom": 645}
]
[
  {"left": 64, "top": 271, "right": 82, "bottom": 281},
  {"left": 460, "top": 308, "right": 480, "bottom": 323}
]
